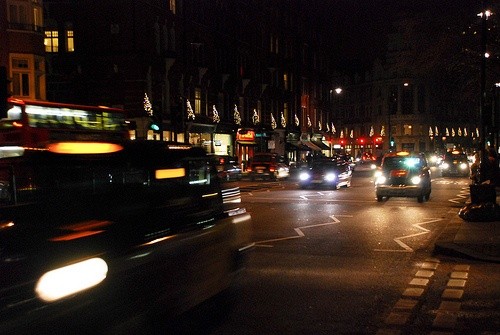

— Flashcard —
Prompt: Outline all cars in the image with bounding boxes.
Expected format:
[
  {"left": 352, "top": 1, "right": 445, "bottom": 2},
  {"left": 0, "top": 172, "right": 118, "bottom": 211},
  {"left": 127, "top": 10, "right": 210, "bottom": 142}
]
[
  {"left": 212, "top": 155, "right": 242, "bottom": 182},
  {"left": 334, "top": 155, "right": 379, "bottom": 178},
  {"left": 0, "top": 120, "right": 251, "bottom": 335},
  {"left": 375, "top": 152, "right": 432, "bottom": 203},
  {"left": 298, "top": 159, "right": 352, "bottom": 190},
  {"left": 247, "top": 153, "right": 289, "bottom": 182},
  {"left": 441, "top": 150, "right": 471, "bottom": 178}
]
